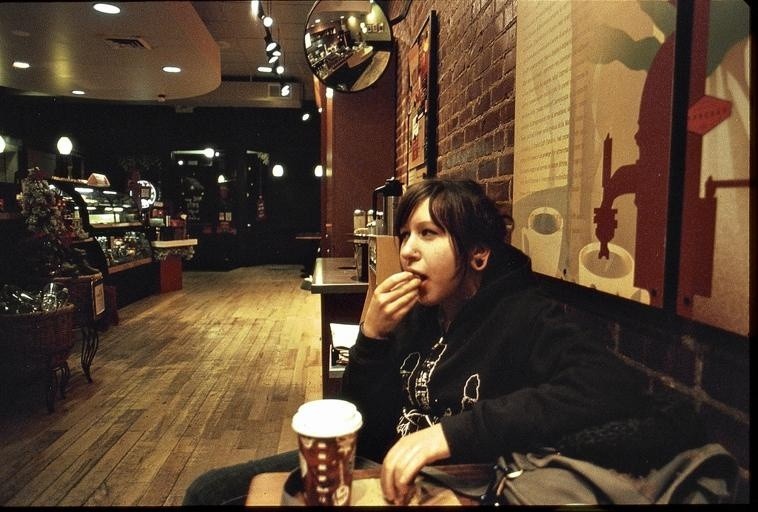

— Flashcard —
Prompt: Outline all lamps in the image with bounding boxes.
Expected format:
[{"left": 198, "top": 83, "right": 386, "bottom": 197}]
[
  {"left": 56, "top": 136, "right": 73, "bottom": 178},
  {"left": 251, "top": 0, "right": 290, "bottom": 97}
]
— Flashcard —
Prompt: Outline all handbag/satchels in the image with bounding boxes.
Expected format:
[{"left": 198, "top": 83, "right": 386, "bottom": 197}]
[{"left": 483, "top": 444, "right": 739, "bottom": 505}]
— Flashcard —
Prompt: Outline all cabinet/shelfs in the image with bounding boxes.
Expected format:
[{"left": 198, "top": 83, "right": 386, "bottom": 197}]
[{"left": 49, "top": 179, "right": 160, "bottom": 310}]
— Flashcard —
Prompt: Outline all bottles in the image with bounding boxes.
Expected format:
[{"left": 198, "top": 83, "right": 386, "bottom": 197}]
[{"left": 1, "top": 283, "right": 70, "bottom": 317}]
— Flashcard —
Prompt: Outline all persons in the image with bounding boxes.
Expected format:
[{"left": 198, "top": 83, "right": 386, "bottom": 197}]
[{"left": 182, "top": 177, "right": 624, "bottom": 506}]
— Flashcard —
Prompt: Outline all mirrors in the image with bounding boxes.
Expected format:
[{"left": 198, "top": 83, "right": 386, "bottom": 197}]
[{"left": 303, "top": 0, "right": 412, "bottom": 95}]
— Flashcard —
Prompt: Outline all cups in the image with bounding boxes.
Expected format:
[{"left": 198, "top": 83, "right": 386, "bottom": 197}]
[
  {"left": 577, "top": 241, "right": 650, "bottom": 303},
  {"left": 521, "top": 208, "right": 566, "bottom": 275},
  {"left": 293, "top": 400, "right": 364, "bottom": 506}
]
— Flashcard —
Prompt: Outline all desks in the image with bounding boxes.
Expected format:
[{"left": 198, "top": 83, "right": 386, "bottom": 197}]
[
  {"left": 311, "top": 257, "right": 370, "bottom": 400},
  {"left": 245, "top": 468, "right": 481, "bottom": 506}
]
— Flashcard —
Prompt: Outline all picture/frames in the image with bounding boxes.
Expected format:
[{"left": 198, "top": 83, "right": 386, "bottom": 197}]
[{"left": 406, "top": 10, "right": 436, "bottom": 189}]
